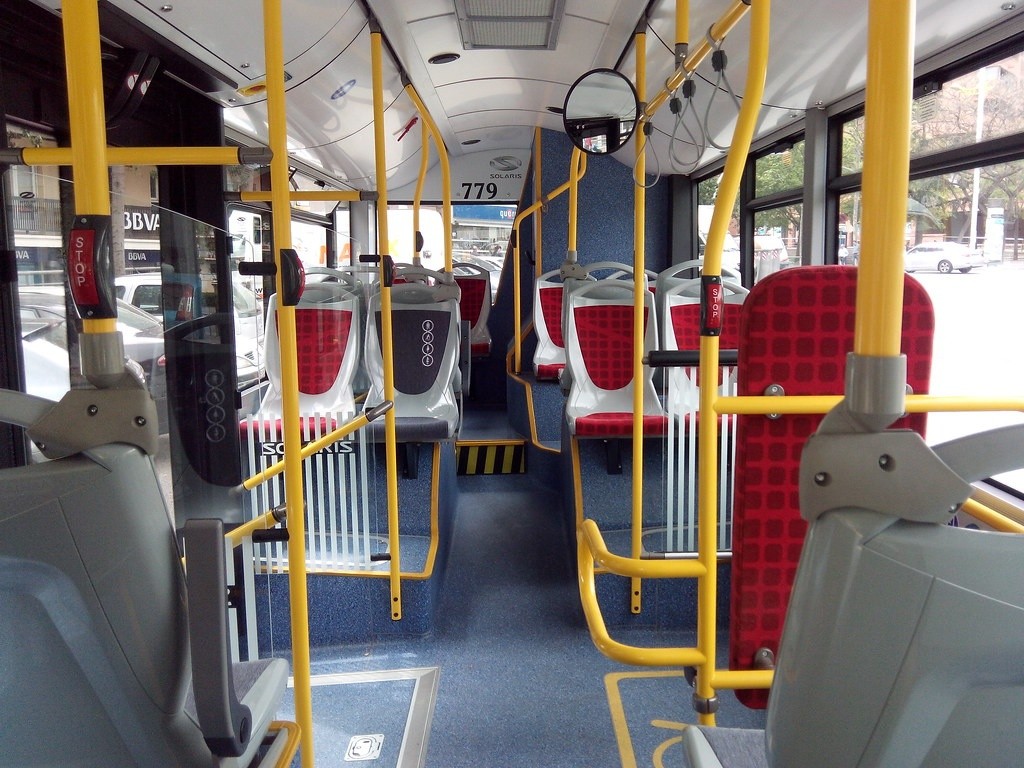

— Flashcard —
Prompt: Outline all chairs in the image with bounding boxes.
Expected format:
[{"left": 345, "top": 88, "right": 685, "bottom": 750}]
[{"left": 0, "top": 252, "right": 1023, "bottom": 768}]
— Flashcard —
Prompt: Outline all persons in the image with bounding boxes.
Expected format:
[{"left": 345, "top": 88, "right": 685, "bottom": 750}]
[
  {"left": 839, "top": 244, "right": 849, "bottom": 265},
  {"left": 472, "top": 246, "right": 479, "bottom": 256},
  {"left": 905, "top": 242, "right": 910, "bottom": 251},
  {"left": 492, "top": 245, "right": 502, "bottom": 256},
  {"left": 853, "top": 240, "right": 859, "bottom": 262}
]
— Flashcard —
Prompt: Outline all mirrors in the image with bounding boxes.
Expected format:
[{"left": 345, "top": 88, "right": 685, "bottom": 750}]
[{"left": 565, "top": 69, "right": 638, "bottom": 155}]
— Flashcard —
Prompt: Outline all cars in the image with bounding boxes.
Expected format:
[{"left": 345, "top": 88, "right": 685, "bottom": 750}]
[
  {"left": 113, "top": 269, "right": 268, "bottom": 380},
  {"left": 448, "top": 239, "right": 513, "bottom": 303},
  {"left": 843, "top": 246, "right": 860, "bottom": 267},
  {"left": 10, "top": 291, "right": 268, "bottom": 429},
  {"left": 696, "top": 230, "right": 740, "bottom": 272},
  {"left": 17, "top": 318, "right": 150, "bottom": 463},
  {"left": 731, "top": 235, "right": 792, "bottom": 268},
  {"left": 900, "top": 240, "right": 985, "bottom": 274}
]
